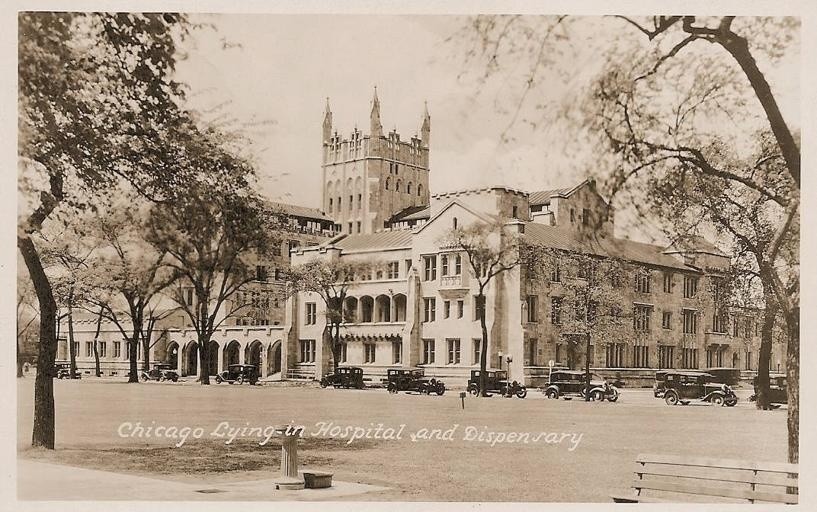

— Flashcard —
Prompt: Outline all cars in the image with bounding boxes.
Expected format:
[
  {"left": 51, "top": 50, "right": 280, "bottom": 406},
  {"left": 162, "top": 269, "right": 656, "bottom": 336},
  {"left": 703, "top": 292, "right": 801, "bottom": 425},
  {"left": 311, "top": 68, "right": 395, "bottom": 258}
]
[
  {"left": 542, "top": 370, "right": 621, "bottom": 403},
  {"left": 53, "top": 362, "right": 82, "bottom": 380},
  {"left": 387, "top": 368, "right": 445, "bottom": 395},
  {"left": 215, "top": 364, "right": 259, "bottom": 385},
  {"left": 754, "top": 374, "right": 788, "bottom": 404},
  {"left": 654, "top": 371, "right": 739, "bottom": 407},
  {"left": 467, "top": 370, "right": 527, "bottom": 398},
  {"left": 320, "top": 366, "right": 366, "bottom": 389},
  {"left": 141, "top": 364, "right": 179, "bottom": 382}
]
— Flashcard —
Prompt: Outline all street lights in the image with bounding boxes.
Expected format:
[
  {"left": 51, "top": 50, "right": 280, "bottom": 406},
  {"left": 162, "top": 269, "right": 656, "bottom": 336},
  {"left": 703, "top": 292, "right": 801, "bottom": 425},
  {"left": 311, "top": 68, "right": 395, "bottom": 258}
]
[
  {"left": 498, "top": 349, "right": 503, "bottom": 370},
  {"left": 777, "top": 360, "right": 780, "bottom": 374}
]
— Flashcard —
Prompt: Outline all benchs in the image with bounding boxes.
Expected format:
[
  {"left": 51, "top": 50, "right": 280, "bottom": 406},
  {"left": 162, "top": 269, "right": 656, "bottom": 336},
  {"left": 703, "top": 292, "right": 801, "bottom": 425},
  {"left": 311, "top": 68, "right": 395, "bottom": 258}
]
[{"left": 609, "top": 452, "right": 799, "bottom": 505}]
